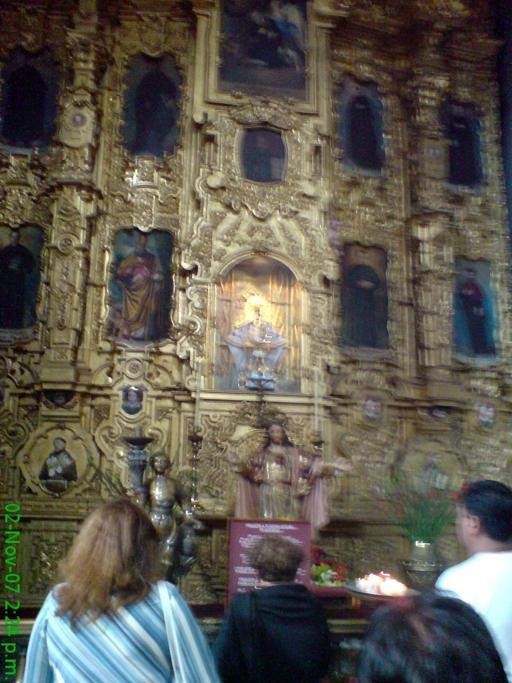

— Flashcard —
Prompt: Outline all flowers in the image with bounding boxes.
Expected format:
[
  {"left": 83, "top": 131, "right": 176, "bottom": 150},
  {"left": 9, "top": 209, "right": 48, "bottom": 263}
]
[{"left": 380, "top": 481, "right": 463, "bottom": 542}]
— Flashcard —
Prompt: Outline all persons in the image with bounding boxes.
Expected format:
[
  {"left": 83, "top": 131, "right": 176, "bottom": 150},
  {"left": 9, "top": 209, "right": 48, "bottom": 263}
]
[
  {"left": 22, "top": 498, "right": 222, "bottom": 683},
  {"left": 130, "top": 57, "right": 177, "bottom": 155},
  {"left": 227, "top": 306, "right": 289, "bottom": 372},
  {"left": 454, "top": 268, "right": 492, "bottom": 354},
  {"left": 211, "top": 534, "right": 331, "bottom": 683},
  {"left": 356, "top": 594, "right": 509, "bottom": 683},
  {"left": 231, "top": 421, "right": 352, "bottom": 520},
  {"left": 342, "top": 250, "right": 388, "bottom": 349},
  {"left": 231, "top": 5, "right": 283, "bottom": 85},
  {"left": 135, "top": 451, "right": 205, "bottom": 580},
  {"left": 2, "top": 53, "right": 49, "bottom": 147},
  {"left": 0, "top": 229, "right": 33, "bottom": 328},
  {"left": 117, "top": 231, "right": 165, "bottom": 342},
  {"left": 434, "top": 480, "right": 512, "bottom": 683},
  {"left": 121, "top": 386, "right": 142, "bottom": 415},
  {"left": 39, "top": 438, "right": 77, "bottom": 493}
]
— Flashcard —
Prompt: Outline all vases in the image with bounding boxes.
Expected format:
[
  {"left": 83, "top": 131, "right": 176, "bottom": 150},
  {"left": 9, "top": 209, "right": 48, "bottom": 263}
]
[{"left": 397, "top": 545, "right": 445, "bottom": 586}]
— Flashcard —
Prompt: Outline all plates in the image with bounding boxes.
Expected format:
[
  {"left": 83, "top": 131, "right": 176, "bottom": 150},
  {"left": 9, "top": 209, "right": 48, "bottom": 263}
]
[{"left": 342, "top": 579, "right": 420, "bottom": 604}]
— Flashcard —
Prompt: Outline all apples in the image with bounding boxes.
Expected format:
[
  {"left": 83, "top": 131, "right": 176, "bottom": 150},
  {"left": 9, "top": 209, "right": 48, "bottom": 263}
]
[{"left": 312, "top": 566, "right": 342, "bottom": 586}]
